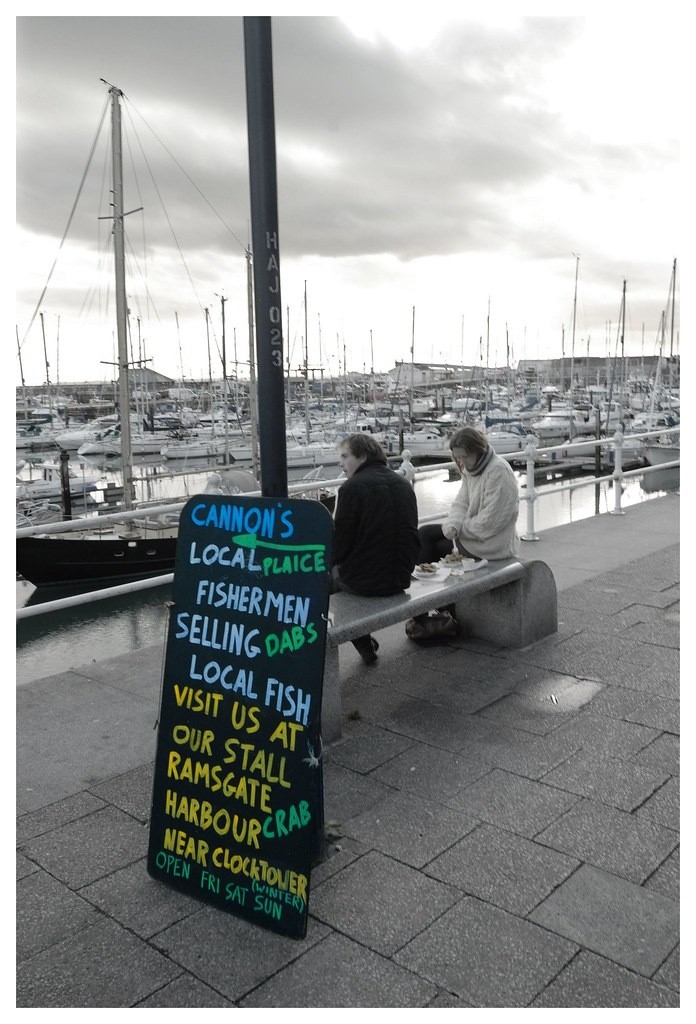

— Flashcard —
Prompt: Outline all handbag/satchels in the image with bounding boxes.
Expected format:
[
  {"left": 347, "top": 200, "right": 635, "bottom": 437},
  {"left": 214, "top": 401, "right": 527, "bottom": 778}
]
[{"left": 406, "top": 611, "right": 458, "bottom": 647}]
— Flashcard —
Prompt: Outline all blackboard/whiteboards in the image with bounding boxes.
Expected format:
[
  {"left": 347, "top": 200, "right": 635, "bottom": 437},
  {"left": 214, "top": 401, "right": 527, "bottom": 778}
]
[{"left": 143, "top": 493, "right": 334, "bottom": 943}]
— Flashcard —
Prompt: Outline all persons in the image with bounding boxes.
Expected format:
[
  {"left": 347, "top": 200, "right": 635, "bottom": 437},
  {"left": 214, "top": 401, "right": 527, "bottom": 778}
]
[
  {"left": 412, "top": 426, "right": 520, "bottom": 620},
  {"left": 330, "top": 434, "right": 423, "bottom": 664}
]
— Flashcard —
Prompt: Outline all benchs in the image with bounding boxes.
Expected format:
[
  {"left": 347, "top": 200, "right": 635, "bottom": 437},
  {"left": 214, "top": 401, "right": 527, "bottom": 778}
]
[{"left": 320, "top": 555, "right": 558, "bottom": 748}]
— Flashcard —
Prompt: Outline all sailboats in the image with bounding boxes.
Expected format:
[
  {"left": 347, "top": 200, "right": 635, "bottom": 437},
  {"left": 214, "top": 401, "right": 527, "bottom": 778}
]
[{"left": 15, "top": 76, "right": 679, "bottom": 583}]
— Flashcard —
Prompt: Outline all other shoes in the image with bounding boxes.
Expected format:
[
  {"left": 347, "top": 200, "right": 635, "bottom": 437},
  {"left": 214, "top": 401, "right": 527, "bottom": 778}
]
[{"left": 360, "top": 636, "right": 379, "bottom": 663}]
